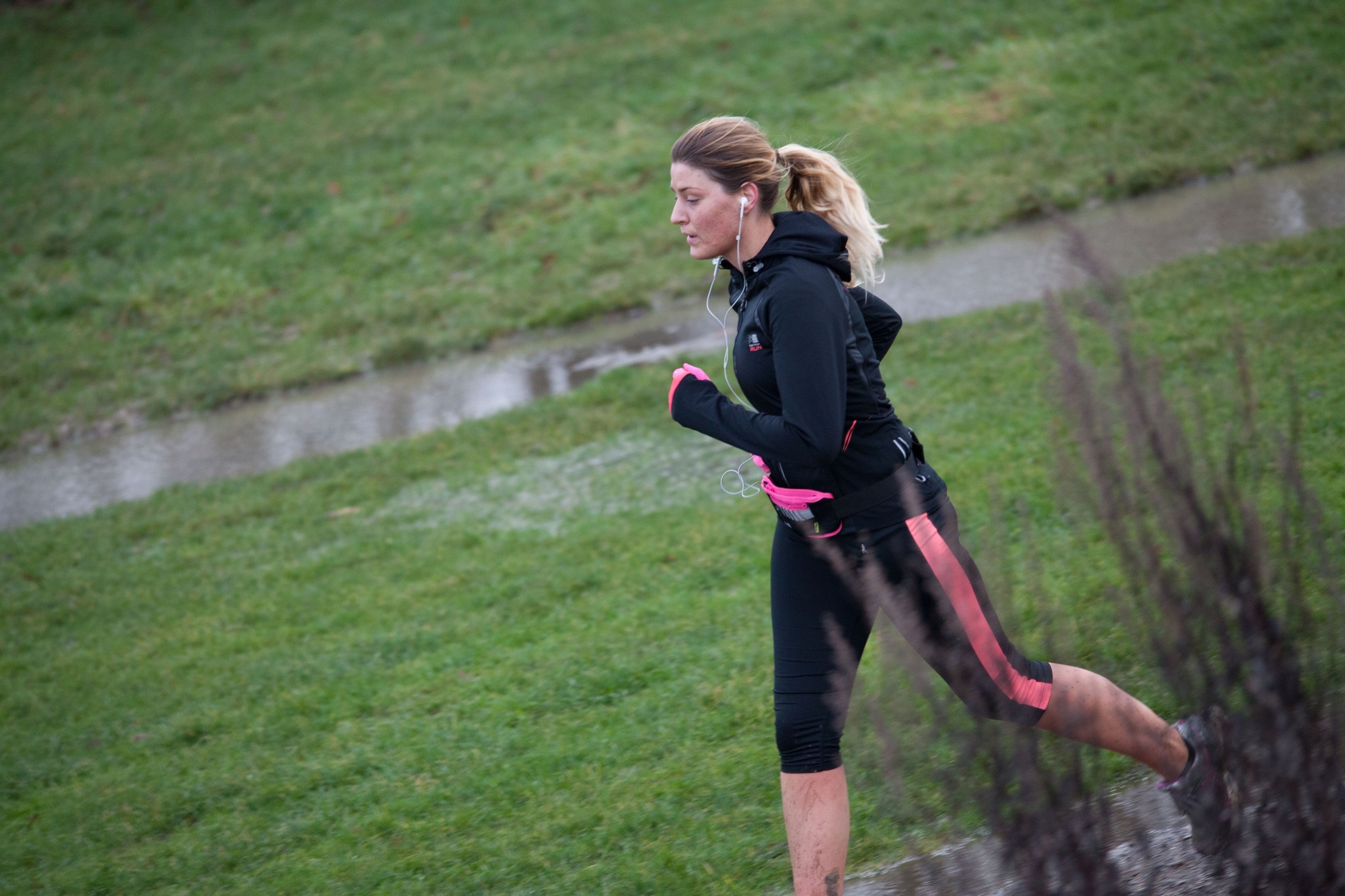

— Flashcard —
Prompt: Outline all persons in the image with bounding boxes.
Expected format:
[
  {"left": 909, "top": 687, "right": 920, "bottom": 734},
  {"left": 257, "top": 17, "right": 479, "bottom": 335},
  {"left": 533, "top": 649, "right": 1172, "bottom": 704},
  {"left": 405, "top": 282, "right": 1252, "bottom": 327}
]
[{"left": 665, "top": 115, "right": 1225, "bottom": 896}]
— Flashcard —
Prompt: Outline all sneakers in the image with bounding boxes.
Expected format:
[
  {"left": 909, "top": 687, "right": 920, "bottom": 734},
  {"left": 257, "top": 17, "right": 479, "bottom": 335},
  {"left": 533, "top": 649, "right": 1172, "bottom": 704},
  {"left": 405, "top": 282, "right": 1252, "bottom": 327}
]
[{"left": 1158, "top": 707, "right": 1236, "bottom": 856}]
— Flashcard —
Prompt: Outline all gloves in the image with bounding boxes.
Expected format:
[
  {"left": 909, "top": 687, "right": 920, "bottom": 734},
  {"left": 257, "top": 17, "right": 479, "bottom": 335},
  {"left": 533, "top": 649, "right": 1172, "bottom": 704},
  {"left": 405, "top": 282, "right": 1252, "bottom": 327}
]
[{"left": 668, "top": 362, "right": 711, "bottom": 416}]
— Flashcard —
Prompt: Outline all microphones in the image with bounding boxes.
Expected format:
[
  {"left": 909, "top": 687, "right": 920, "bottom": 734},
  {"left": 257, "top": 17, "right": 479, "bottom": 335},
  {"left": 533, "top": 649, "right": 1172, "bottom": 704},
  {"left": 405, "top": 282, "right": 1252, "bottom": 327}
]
[{"left": 714, "top": 258, "right": 721, "bottom": 277}]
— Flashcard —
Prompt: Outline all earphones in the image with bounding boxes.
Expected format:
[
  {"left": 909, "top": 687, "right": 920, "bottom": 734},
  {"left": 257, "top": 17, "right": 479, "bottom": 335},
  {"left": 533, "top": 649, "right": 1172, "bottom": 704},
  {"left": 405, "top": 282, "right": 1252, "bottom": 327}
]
[{"left": 739, "top": 198, "right": 749, "bottom": 218}]
